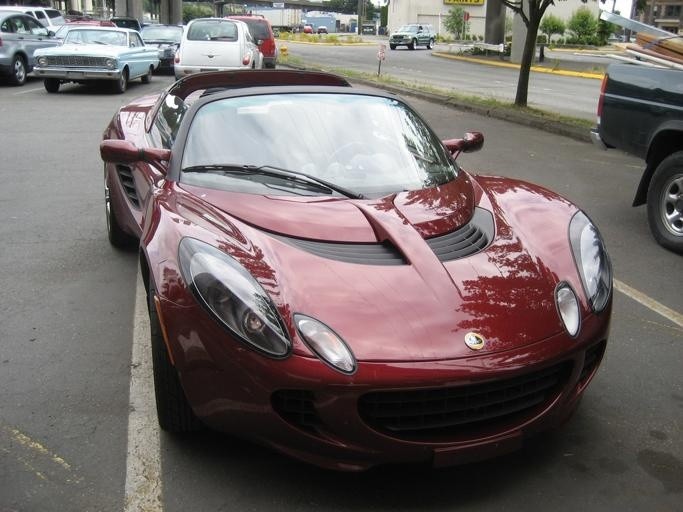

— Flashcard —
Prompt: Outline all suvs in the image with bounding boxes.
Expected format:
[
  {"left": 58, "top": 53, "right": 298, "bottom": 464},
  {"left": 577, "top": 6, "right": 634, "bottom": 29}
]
[{"left": 388, "top": 23, "right": 435, "bottom": 51}]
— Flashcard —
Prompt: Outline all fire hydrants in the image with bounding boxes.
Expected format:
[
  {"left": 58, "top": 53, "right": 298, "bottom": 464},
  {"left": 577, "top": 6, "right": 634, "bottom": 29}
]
[{"left": 277, "top": 44, "right": 289, "bottom": 65}]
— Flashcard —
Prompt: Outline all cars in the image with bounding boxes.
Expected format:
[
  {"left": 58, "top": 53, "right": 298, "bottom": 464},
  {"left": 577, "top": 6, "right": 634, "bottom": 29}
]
[
  {"left": 0, "top": 3, "right": 278, "bottom": 91},
  {"left": 301, "top": 25, "right": 331, "bottom": 35}
]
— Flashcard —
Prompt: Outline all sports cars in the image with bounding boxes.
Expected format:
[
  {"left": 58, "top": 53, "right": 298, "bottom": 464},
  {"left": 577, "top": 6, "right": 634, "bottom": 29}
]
[{"left": 99, "top": 65, "right": 613, "bottom": 488}]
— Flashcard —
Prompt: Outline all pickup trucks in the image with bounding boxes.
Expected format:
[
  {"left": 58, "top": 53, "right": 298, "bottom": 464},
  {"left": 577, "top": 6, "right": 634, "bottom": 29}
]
[{"left": 592, "top": 60, "right": 682, "bottom": 259}]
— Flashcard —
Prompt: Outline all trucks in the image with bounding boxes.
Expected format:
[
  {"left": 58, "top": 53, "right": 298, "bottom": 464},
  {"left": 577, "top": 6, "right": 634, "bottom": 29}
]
[{"left": 248, "top": 6, "right": 316, "bottom": 34}]
